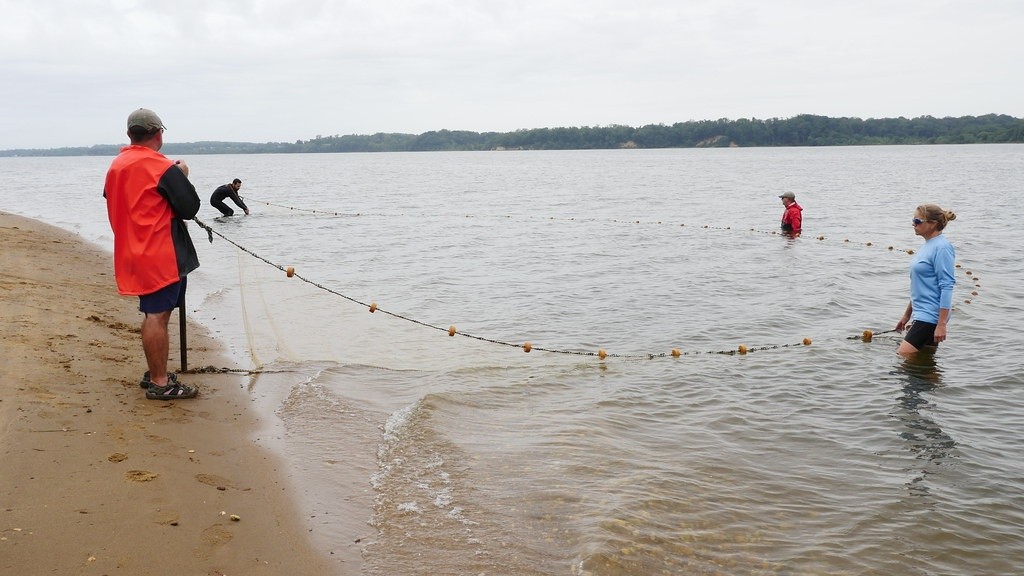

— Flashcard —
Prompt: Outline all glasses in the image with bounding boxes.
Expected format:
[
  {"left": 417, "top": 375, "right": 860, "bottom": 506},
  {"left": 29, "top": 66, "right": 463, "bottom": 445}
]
[{"left": 911, "top": 218, "right": 932, "bottom": 224}]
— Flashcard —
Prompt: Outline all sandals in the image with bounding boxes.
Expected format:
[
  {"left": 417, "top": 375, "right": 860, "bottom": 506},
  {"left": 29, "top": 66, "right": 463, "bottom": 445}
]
[
  {"left": 140, "top": 370, "right": 177, "bottom": 388},
  {"left": 146, "top": 381, "right": 198, "bottom": 400}
]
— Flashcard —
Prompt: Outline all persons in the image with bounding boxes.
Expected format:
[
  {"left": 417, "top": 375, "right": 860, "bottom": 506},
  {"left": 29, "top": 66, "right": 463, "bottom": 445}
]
[
  {"left": 102, "top": 108, "right": 198, "bottom": 399},
  {"left": 210, "top": 178, "right": 250, "bottom": 218},
  {"left": 779, "top": 192, "right": 803, "bottom": 237},
  {"left": 895, "top": 205, "right": 957, "bottom": 357}
]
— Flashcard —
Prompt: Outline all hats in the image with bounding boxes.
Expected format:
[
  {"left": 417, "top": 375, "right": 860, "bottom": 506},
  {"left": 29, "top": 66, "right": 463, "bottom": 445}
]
[
  {"left": 779, "top": 191, "right": 795, "bottom": 198},
  {"left": 127, "top": 108, "right": 167, "bottom": 133}
]
